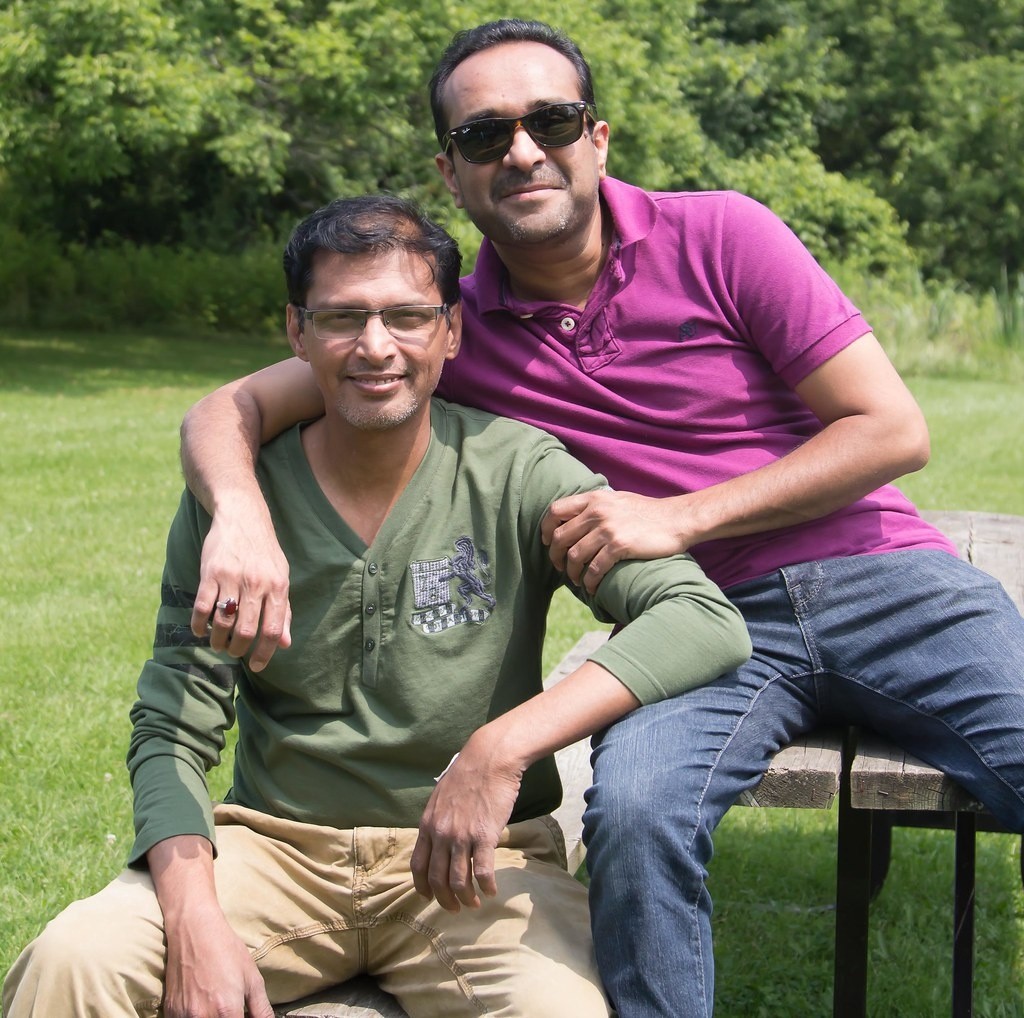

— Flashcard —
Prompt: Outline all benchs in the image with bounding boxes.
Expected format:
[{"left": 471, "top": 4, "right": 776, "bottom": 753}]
[{"left": 248, "top": 632, "right": 621, "bottom": 1018}]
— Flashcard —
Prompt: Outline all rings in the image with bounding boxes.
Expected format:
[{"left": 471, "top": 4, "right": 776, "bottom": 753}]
[{"left": 217, "top": 598, "right": 238, "bottom": 616}]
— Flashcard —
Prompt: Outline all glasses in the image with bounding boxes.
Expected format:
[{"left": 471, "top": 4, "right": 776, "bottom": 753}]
[
  {"left": 293, "top": 303, "right": 450, "bottom": 341},
  {"left": 443, "top": 100, "right": 599, "bottom": 164}
]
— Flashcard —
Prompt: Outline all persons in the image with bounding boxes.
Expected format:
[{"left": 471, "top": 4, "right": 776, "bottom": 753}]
[
  {"left": 1, "top": 196, "right": 752, "bottom": 1018},
  {"left": 179, "top": 15, "right": 1024, "bottom": 1018}
]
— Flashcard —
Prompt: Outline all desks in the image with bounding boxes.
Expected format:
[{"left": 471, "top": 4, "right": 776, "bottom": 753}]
[{"left": 619, "top": 509, "right": 1023, "bottom": 1016}]
